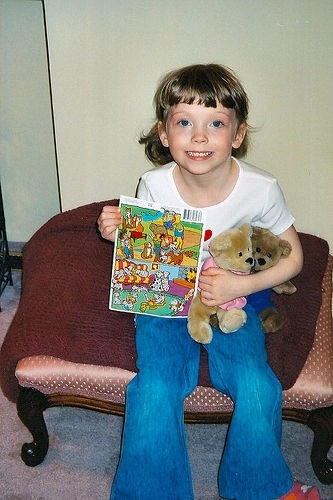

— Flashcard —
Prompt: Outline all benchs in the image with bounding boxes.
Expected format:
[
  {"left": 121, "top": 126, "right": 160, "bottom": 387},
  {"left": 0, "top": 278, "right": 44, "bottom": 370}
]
[{"left": 0, "top": 198, "right": 333, "bottom": 486}]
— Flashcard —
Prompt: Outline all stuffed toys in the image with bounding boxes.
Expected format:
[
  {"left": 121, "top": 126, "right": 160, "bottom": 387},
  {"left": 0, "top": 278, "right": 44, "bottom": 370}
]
[
  {"left": 209, "top": 225, "right": 298, "bottom": 332},
  {"left": 187, "top": 223, "right": 253, "bottom": 343}
]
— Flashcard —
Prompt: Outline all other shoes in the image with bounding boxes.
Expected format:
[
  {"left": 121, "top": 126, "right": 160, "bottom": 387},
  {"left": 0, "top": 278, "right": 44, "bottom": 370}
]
[{"left": 278, "top": 479, "right": 320, "bottom": 500}]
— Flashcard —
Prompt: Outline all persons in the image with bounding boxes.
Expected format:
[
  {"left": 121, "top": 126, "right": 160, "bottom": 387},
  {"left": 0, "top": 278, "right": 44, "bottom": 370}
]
[{"left": 98, "top": 63, "right": 320, "bottom": 500}]
